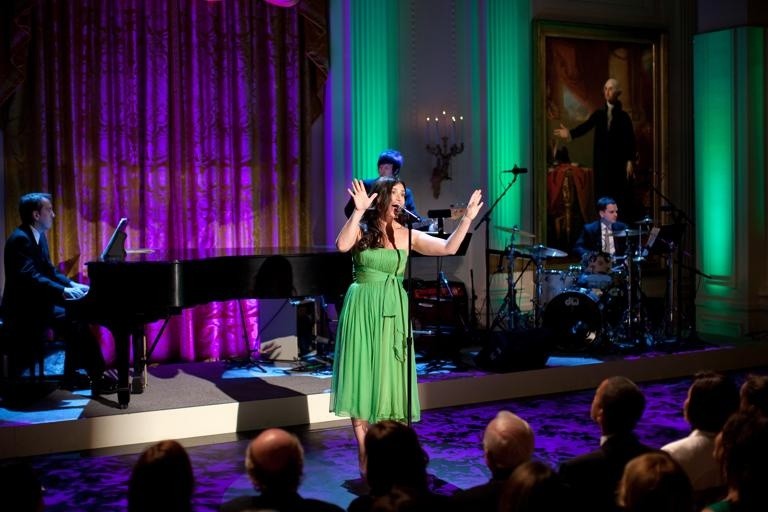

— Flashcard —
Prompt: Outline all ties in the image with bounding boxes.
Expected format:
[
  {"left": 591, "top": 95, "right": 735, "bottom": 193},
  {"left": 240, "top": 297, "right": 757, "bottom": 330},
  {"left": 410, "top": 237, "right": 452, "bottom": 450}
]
[{"left": 604, "top": 224, "right": 611, "bottom": 253}]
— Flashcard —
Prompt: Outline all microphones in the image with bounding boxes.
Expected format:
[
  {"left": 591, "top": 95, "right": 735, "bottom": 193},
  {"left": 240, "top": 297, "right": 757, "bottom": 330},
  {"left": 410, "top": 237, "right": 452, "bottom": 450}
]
[
  {"left": 511, "top": 165, "right": 529, "bottom": 176},
  {"left": 436, "top": 267, "right": 455, "bottom": 296},
  {"left": 398, "top": 203, "right": 423, "bottom": 226},
  {"left": 637, "top": 217, "right": 654, "bottom": 228}
]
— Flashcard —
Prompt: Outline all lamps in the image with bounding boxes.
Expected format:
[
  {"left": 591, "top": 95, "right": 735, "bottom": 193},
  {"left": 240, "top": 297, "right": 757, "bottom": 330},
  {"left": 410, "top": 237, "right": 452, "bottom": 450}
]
[{"left": 425, "top": 108, "right": 466, "bottom": 200}]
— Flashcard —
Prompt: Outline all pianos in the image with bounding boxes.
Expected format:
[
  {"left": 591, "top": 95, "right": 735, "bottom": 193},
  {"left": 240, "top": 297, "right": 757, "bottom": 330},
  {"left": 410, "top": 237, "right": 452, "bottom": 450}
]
[{"left": 63, "top": 246, "right": 354, "bottom": 409}]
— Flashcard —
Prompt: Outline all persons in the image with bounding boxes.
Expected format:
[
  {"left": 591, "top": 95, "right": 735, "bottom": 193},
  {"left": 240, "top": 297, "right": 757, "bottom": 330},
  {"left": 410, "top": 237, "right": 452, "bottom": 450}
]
[
  {"left": 346, "top": 421, "right": 451, "bottom": 512},
  {"left": 452, "top": 369, "right": 768, "bottom": 512},
  {"left": 573, "top": 196, "right": 646, "bottom": 328},
  {"left": 345, "top": 150, "right": 439, "bottom": 232},
  {"left": 128, "top": 440, "right": 197, "bottom": 512},
  {"left": 1, "top": 456, "right": 46, "bottom": 512},
  {"left": 553, "top": 78, "right": 644, "bottom": 230},
  {"left": 215, "top": 427, "right": 344, "bottom": 512},
  {"left": 328, "top": 178, "right": 484, "bottom": 477},
  {"left": 2, "top": 192, "right": 120, "bottom": 395}
]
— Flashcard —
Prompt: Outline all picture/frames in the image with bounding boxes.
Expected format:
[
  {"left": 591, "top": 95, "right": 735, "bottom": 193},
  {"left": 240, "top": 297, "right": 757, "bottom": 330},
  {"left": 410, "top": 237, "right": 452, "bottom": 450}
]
[{"left": 533, "top": 20, "right": 675, "bottom": 277}]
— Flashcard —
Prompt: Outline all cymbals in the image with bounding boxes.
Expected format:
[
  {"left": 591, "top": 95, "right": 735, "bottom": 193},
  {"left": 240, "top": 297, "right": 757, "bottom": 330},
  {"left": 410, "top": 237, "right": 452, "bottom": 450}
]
[
  {"left": 634, "top": 219, "right": 658, "bottom": 224},
  {"left": 508, "top": 245, "right": 568, "bottom": 260},
  {"left": 603, "top": 230, "right": 647, "bottom": 239},
  {"left": 495, "top": 225, "right": 537, "bottom": 238}
]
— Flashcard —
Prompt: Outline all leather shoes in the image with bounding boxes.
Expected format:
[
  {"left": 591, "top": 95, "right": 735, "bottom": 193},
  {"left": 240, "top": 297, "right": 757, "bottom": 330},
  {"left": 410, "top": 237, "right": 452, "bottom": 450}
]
[{"left": 91, "top": 376, "right": 132, "bottom": 397}]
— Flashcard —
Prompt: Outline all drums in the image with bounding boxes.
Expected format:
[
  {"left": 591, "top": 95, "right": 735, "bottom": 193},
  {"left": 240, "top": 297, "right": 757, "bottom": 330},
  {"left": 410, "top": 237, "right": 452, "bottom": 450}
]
[
  {"left": 541, "top": 269, "right": 574, "bottom": 312},
  {"left": 541, "top": 288, "right": 606, "bottom": 355},
  {"left": 576, "top": 251, "right": 613, "bottom": 289}
]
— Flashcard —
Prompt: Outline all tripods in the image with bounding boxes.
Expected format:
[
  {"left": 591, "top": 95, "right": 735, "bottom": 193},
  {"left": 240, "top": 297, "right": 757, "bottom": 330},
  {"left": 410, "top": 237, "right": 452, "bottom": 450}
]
[
  {"left": 483, "top": 266, "right": 525, "bottom": 331},
  {"left": 606, "top": 279, "right": 704, "bottom": 348}
]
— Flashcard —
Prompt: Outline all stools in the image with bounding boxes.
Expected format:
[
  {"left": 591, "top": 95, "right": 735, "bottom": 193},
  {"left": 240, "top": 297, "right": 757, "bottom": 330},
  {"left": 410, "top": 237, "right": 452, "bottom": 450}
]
[{"left": 0, "top": 326, "right": 57, "bottom": 399}]
